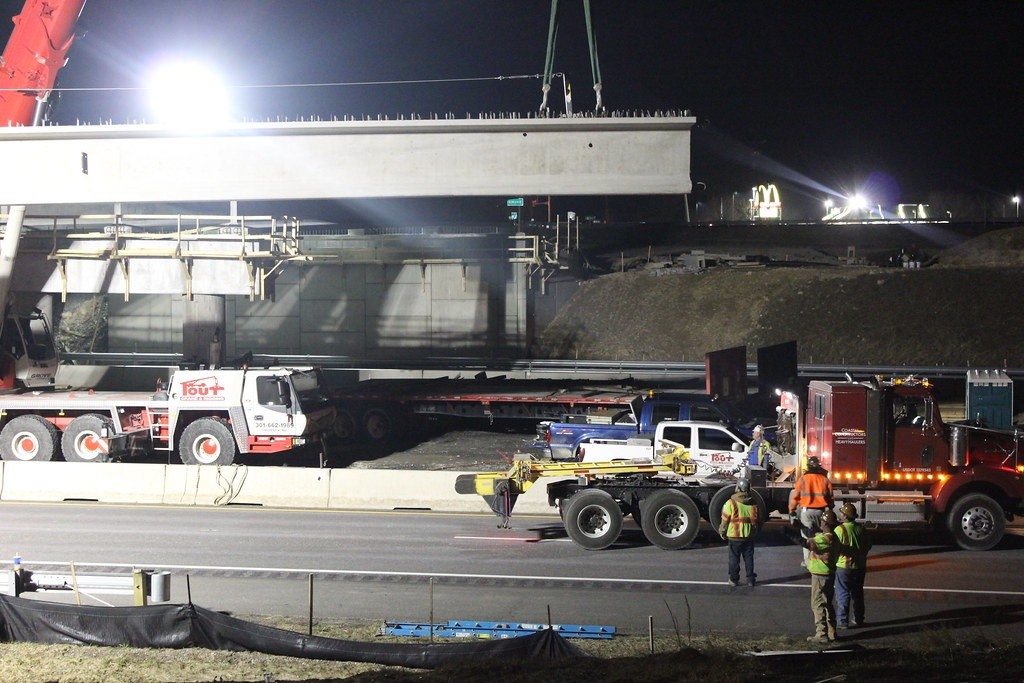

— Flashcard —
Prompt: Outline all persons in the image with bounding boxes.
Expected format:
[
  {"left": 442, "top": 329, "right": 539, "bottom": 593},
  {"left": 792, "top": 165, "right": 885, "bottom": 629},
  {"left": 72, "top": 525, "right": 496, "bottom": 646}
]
[
  {"left": 718, "top": 479, "right": 761, "bottom": 587},
  {"left": 810, "top": 502, "right": 873, "bottom": 630},
  {"left": 748, "top": 424, "right": 773, "bottom": 521},
  {"left": 788, "top": 456, "right": 837, "bottom": 567},
  {"left": 777, "top": 510, "right": 841, "bottom": 645}
]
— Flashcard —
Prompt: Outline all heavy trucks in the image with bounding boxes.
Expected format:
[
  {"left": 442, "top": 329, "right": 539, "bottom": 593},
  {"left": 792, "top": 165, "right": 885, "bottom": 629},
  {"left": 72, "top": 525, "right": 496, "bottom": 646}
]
[
  {"left": 191, "top": 341, "right": 803, "bottom": 445},
  {"left": 0, "top": 352, "right": 342, "bottom": 468},
  {"left": 453, "top": 365, "right": 1024, "bottom": 557}
]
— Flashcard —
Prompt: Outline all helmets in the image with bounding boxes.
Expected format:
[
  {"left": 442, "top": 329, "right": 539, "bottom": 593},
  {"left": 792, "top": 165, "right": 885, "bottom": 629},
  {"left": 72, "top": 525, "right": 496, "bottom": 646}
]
[
  {"left": 839, "top": 505, "right": 859, "bottom": 519},
  {"left": 821, "top": 511, "right": 840, "bottom": 525},
  {"left": 807, "top": 457, "right": 819, "bottom": 467},
  {"left": 737, "top": 479, "right": 750, "bottom": 492},
  {"left": 752, "top": 425, "right": 764, "bottom": 434}
]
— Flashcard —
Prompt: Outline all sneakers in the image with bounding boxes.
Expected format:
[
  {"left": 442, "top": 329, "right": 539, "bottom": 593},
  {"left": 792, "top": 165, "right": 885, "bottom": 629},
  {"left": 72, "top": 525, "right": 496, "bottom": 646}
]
[
  {"left": 835, "top": 623, "right": 848, "bottom": 629},
  {"left": 851, "top": 618, "right": 863, "bottom": 625}
]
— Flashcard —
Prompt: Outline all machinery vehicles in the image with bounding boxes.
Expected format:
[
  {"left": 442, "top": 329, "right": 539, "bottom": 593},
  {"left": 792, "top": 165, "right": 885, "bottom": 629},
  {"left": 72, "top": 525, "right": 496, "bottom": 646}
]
[{"left": 2, "top": 0, "right": 107, "bottom": 392}]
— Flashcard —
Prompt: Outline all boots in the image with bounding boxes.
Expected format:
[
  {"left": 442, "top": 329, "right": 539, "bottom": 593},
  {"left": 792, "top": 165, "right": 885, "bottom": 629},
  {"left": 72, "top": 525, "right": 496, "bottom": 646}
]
[
  {"left": 807, "top": 631, "right": 829, "bottom": 644},
  {"left": 827, "top": 627, "right": 837, "bottom": 641}
]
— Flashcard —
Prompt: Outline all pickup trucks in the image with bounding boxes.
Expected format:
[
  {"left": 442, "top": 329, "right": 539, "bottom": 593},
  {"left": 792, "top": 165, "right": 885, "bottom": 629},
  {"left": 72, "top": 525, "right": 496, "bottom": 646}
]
[
  {"left": 542, "top": 389, "right": 786, "bottom": 468},
  {"left": 579, "top": 423, "right": 755, "bottom": 480}
]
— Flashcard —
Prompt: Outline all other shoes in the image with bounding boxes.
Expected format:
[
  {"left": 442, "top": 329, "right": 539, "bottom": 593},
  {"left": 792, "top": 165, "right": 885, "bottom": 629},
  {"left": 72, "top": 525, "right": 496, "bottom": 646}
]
[
  {"left": 801, "top": 560, "right": 806, "bottom": 566},
  {"left": 747, "top": 581, "right": 754, "bottom": 586},
  {"left": 729, "top": 579, "right": 737, "bottom": 586}
]
